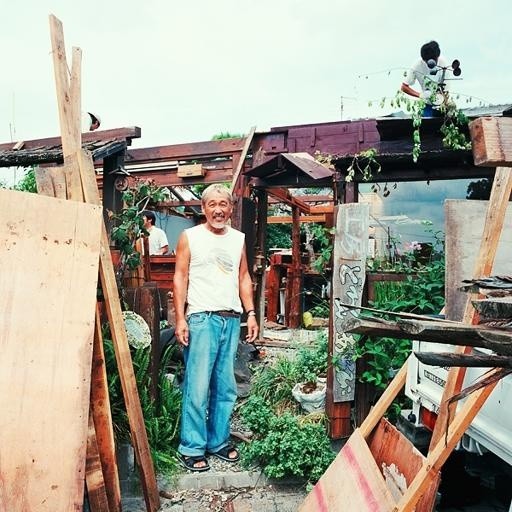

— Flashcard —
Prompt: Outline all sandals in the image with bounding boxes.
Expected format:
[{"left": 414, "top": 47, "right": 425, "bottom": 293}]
[
  {"left": 206, "top": 445, "right": 240, "bottom": 461},
  {"left": 176, "top": 452, "right": 209, "bottom": 471}
]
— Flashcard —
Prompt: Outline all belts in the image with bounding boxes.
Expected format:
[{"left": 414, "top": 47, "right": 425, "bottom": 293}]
[{"left": 206, "top": 310, "right": 241, "bottom": 318}]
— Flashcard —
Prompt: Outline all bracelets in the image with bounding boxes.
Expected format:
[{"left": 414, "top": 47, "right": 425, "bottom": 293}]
[
  {"left": 248, "top": 313, "right": 255, "bottom": 317},
  {"left": 246, "top": 309, "right": 256, "bottom": 314}
]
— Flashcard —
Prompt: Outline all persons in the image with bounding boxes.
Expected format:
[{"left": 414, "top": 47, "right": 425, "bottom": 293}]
[
  {"left": 173, "top": 184, "right": 260, "bottom": 472},
  {"left": 401, "top": 41, "right": 452, "bottom": 117},
  {"left": 134, "top": 211, "right": 169, "bottom": 257}
]
